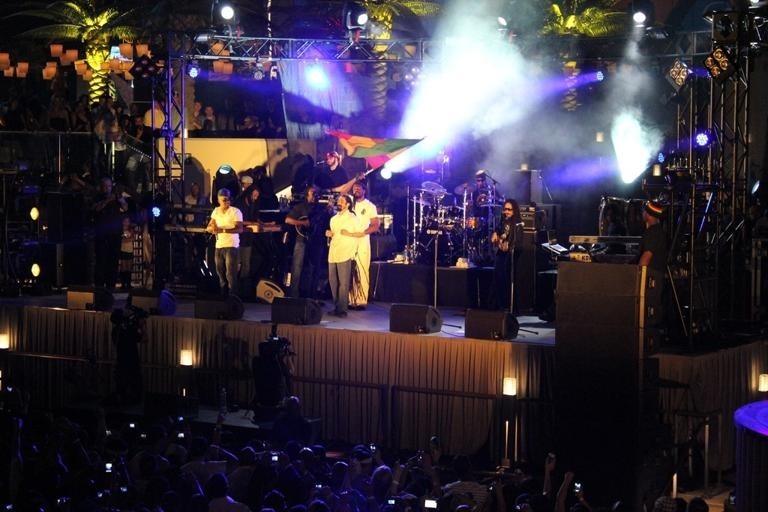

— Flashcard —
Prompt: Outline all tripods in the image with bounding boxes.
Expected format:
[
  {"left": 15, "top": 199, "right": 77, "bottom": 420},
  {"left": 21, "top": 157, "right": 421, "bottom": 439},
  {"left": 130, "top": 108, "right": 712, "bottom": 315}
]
[
  {"left": 508, "top": 227, "right": 538, "bottom": 334},
  {"left": 434, "top": 235, "right": 462, "bottom": 328}
]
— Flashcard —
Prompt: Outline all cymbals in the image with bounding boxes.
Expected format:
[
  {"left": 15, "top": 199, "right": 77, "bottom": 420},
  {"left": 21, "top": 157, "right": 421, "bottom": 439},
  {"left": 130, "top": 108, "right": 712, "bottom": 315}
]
[
  {"left": 454, "top": 184, "right": 477, "bottom": 195},
  {"left": 422, "top": 181, "right": 446, "bottom": 198}
]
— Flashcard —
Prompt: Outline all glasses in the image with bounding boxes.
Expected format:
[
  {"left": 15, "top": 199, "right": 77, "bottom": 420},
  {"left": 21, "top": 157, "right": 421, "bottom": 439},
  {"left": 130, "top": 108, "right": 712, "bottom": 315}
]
[{"left": 503, "top": 208, "right": 513, "bottom": 211}]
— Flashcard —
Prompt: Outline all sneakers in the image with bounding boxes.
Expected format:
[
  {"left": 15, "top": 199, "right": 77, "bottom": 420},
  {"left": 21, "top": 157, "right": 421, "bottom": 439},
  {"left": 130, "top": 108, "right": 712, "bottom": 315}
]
[
  {"left": 347, "top": 305, "right": 365, "bottom": 311},
  {"left": 327, "top": 309, "right": 347, "bottom": 318}
]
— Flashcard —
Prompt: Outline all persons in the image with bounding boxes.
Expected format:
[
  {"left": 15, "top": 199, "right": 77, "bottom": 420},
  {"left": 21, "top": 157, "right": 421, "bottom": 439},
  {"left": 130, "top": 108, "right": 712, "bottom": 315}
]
[
  {"left": 110, "top": 306, "right": 149, "bottom": 410},
  {"left": 1, "top": 82, "right": 523, "bottom": 319},
  {"left": 267, "top": 337, "right": 296, "bottom": 401},
  {"left": 636, "top": 200, "right": 668, "bottom": 353},
  {"left": 602, "top": 204, "right": 627, "bottom": 254},
  {"left": 1, "top": 383, "right": 710, "bottom": 512},
  {"left": 250, "top": 341, "right": 275, "bottom": 408}
]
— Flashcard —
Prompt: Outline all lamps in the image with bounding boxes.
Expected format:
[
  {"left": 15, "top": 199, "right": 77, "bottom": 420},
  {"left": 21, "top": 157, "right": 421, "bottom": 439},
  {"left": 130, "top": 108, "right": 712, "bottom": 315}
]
[
  {"left": 345, "top": 2, "right": 368, "bottom": 28},
  {"left": 496, "top": 10, "right": 514, "bottom": 26},
  {"left": 184, "top": 58, "right": 200, "bottom": 79},
  {"left": 502, "top": 376, "right": 517, "bottom": 398},
  {"left": 179, "top": 348, "right": 193, "bottom": 368},
  {"left": 632, "top": 9, "right": 647, "bottom": 29},
  {"left": 28, "top": 263, "right": 40, "bottom": 278},
  {"left": 749, "top": 179, "right": 766, "bottom": 201},
  {"left": 220, "top": 6, "right": 235, "bottom": 24},
  {"left": 24, "top": 206, "right": 42, "bottom": 222},
  {"left": 756, "top": 373, "right": 767, "bottom": 393},
  {"left": 663, "top": 0, "right": 767, "bottom": 93},
  {"left": 0, "top": 332, "right": 10, "bottom": 350},
  {"left": 695, "top": 131, "right": 713, "bottom": 147}
]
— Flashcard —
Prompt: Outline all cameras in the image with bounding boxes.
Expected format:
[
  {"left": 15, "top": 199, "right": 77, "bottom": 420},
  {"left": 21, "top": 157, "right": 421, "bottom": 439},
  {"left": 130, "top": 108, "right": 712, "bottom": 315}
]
[{"left": 112, "top": 185, "right": 125, "bottom": 200}]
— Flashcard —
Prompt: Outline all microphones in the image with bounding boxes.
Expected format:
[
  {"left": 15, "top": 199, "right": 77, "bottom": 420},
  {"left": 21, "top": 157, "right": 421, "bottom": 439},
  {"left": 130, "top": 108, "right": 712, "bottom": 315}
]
[{"left": 326, "top": 230, "right": 330, "bottom": 247}]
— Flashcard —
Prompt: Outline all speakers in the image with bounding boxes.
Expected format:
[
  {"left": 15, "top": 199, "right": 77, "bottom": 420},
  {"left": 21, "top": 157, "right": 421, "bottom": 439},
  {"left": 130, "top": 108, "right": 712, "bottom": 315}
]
[
  {"left": 194, "top": 294, "right": 244, "bottom": 320},
  {"left": 465, "top": 307, "right": 519, "bottom": 340},
  {"left": 271, "top": 296, "right": 322, "bottom": 325},
  {"left": 389, "top": 303, "right": 442, "bottom": 333},
  {"left": 129, "top": 288, "right": 177, "bottom": 315},
  {"left": 66, "top": 286, "right": 116, "bottom": 309}
]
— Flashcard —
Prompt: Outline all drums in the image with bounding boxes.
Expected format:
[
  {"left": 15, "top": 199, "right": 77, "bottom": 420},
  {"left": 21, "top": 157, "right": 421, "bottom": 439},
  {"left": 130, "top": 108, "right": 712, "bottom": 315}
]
[
  {"left": 421, "top": 205, "right": 459, "bottom": 238},
  {"left": 462, "top": 217, "right": 484, "bottom": 233}
]
